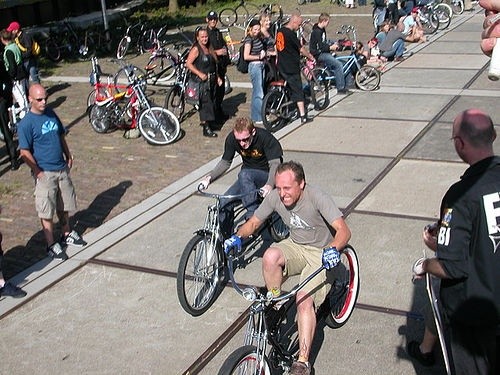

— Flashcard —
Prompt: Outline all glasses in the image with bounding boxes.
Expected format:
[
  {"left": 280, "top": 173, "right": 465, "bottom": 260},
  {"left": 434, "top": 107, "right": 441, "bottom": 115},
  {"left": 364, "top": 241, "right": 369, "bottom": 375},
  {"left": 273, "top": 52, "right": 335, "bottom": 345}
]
[
  {"left": 235, "top": 135, "right": 251, "bottom": 142},
  {"left": 35, "top": 95, "right": 48, "bottom": 102}
]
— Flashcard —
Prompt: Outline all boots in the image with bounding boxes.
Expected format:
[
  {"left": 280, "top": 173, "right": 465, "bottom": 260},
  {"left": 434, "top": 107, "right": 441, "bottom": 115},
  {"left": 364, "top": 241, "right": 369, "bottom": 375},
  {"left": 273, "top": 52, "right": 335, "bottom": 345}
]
[{"left": 202, "top": 122, "right": 217, "bottom": 137}]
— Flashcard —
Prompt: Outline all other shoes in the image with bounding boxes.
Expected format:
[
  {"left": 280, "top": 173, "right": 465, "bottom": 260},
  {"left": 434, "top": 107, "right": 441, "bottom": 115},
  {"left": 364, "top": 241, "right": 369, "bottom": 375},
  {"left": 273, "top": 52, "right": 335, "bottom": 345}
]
[
  {"left": 0, "top": 281, "right": 27, "bottom": 298},
  {"left": 300, "top": 112, "right": 313, "bottom": 125},
  {"left": 407, "top": 340, "right": 436, "bottom": 367},
  {"left": 337, "top": 88, "right": 353, "bottom": 96}
]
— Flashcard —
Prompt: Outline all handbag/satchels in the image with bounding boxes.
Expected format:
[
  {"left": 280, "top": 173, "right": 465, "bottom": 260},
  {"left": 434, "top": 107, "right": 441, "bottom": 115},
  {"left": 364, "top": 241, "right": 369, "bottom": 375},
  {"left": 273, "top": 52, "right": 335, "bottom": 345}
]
[
  {"left": 237, "top": 40, "right": 254, "bottom": 74},
  {"left": 32, "top": 40, "right": 40, "bottom": 55},
  {"left": 185, "top": 78, "right": 202, "bottom": 105}
]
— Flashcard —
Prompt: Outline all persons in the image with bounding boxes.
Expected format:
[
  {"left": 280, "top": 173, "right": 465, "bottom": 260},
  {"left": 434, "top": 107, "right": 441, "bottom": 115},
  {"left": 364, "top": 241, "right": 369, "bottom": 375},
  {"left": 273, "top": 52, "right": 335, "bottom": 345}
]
[
  {"left": 310, "top": 12, "right": 352, "bottom": 95},
  {"left": 17, "top": 84, "right": 87, "bottom": 260},
  {"left": 276, "top": 15, "right": 313, "bottom": 123},
  {"left": 346, "top": 0, "right": 366, "bottom": 8},
  {"left": 224, "top": 161, "right": 351, "bottom": 375},
  {"left": 186, "top": 28, "right": 223, "bottom": 137},
  {"left": 243, "top": 19, "right": 270, "bottom": 124},
  {"left": 0, "top": 22, "right": 40, "bottom": 120},
  {"left": 349, "top": 37, "right": 385, "bottom": 83},
  {"left": 260, "top": 13, "right": 278, "bottom": 91},
  {"left": 0, "top": 231, "right": 27, "bottom": 297},
  {"left": 376, "top": 21, "right": 415, "bottom": 61},
  {"left": 479, "top": 0, "right": 500, "bottom": 56},
  {"left": 374, "top": 0, "right": 430, "bottom": 42},
  {"left": 206, "top": 11, "right": 231, "bottom": 119},
  {"left": 407, "top": 110, "right": 500, "bottom": 365},
  {"left": 197, "top": 117, "right": 284, "bottom": 259}
]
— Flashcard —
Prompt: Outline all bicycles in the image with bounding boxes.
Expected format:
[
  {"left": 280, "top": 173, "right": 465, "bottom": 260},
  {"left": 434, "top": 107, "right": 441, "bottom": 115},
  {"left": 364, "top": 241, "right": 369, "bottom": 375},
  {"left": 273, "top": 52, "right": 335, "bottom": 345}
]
[
  {"left": 217, "top": 240, "right": 361, "bottom": 375},
  {"left": 372, "top": 0, "right": 465, "bottom": 34},
  {"left": 42, "top": 12, "right": 171, "bottom": 62},
  {"left": 85, "top": 1, "right": 381, "bottom": 146},
  {"left": 176, "top": 184, "right": 290, "bottom": 318}
]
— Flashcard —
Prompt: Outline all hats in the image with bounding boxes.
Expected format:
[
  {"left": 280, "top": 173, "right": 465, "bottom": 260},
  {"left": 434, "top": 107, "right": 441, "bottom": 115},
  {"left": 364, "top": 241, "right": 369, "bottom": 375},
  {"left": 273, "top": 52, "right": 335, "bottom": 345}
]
[
  {"left": 6, "top": 22, "right": 20, "bottom": 33},
  {"left": 249, "top": 19, "right": 261, "bottom": 26},
  {"left": 411, "top": 8, "right": 418, "bottom": 13},
  {"left": 207, "top": 10, "right": 218, "bottom": 18}
]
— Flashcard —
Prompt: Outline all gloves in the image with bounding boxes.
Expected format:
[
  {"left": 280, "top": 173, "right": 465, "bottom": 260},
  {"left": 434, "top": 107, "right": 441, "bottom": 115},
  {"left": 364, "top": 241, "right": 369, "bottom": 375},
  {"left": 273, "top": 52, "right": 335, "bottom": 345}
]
[
  {"left": 224, "top": 236, "right": 242, "bottom": 255},
  {"left": 322, "top": 247, "right": 341, "bottom": 270}
]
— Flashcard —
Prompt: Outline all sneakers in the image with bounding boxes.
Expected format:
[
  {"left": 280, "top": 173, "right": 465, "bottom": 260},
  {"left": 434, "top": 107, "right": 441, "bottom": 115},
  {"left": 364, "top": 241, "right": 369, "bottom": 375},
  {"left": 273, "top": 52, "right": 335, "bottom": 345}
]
[
  {"left": 59, "top": 229, "right": 87, "bottom": 247},
  {"left": 288, "top": 360, "right": 312, "bottom": 375},
  {"left": 46, "top": 241, "right": 68, "bottom": 261}
]
